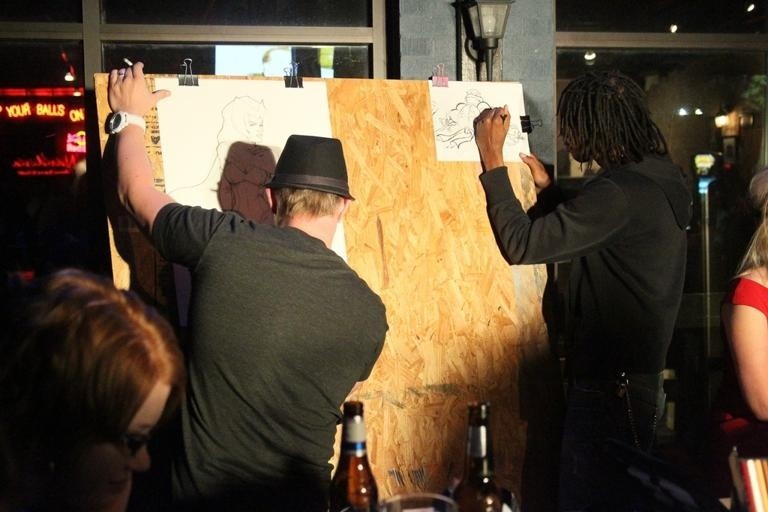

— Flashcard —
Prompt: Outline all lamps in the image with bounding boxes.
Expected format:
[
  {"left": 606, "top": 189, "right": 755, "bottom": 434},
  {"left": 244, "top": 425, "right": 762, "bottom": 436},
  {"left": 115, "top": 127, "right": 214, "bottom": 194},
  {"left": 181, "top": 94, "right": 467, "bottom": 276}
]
[{"left": 451, "top": 0, "right": 516, "bottom": 79}]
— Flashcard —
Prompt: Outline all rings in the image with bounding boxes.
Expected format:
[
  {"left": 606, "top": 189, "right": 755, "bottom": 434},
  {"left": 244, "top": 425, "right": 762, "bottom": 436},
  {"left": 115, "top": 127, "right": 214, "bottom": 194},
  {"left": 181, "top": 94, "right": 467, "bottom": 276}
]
[{"left": 118, "top": 72, "right": 126, "bottom": 76}]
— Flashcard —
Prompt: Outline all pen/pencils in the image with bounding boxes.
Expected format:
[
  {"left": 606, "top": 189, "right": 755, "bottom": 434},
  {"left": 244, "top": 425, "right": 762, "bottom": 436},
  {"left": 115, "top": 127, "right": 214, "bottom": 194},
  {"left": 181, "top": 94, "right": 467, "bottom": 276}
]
[{"left": 503, "top": 115, "right": 507, "bottom": 120}]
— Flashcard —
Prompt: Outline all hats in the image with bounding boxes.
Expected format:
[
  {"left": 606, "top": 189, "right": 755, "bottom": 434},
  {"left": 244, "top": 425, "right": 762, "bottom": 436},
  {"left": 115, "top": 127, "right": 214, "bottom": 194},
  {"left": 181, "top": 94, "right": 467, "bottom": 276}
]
[{"left": 262, "top": 134, "right": 357, "bottom": 200}]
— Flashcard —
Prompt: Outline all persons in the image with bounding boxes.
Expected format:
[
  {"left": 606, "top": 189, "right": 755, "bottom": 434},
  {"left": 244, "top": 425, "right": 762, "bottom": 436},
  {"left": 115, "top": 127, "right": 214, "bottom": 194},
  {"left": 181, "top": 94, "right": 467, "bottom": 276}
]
[
  {"left": 472, "top": 58, "right": 696, "bottom": 512},
  {"left": 0, "top": 265, "right": 183, "bottom": 511},
  {"left": 106, "top": 55, "right": 389, "bottom": 508},
  {"left": 720, "top": 166, "right": 768, "bottom": 511}
]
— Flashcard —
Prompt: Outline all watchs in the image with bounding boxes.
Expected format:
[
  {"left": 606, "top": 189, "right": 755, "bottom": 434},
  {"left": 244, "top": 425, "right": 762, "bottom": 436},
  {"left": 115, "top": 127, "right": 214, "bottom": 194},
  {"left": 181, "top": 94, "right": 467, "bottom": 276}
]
[{"left": 108, "top": 106, "right": 151, "bottom": 137}]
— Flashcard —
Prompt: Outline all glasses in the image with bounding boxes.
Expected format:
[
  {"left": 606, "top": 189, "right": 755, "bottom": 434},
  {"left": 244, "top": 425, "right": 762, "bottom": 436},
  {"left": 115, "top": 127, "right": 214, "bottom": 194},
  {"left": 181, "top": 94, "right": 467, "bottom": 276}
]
[{"left": 125, "top": 431, "right": 150, "bottom": 453}]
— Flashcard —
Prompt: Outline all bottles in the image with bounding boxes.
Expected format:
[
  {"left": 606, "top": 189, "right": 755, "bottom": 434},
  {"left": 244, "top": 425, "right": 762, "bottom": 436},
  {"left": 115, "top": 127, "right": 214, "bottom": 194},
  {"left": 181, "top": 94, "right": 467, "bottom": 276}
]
[
  {"left": 331, "top": 400, "right": 381, "bottom": 511},
  {"left": 727, "top": 429, "right": 768, "bottom": 512},
  {"left": 453, "top": 402, "right": 504, "bottom": 512}
]
